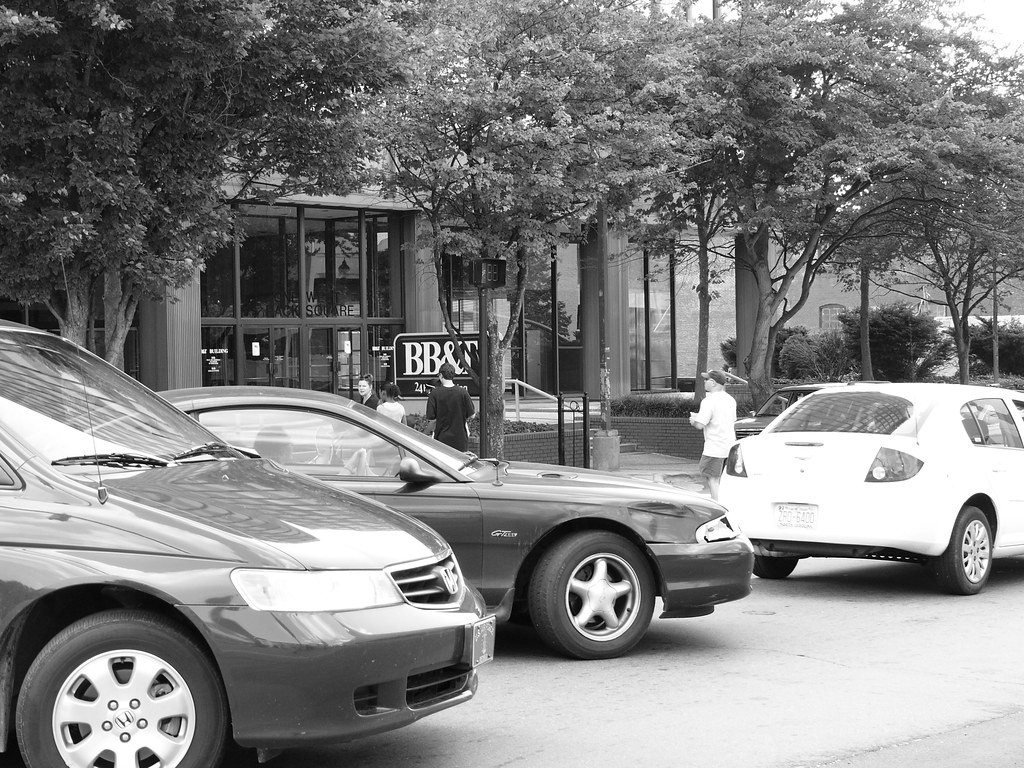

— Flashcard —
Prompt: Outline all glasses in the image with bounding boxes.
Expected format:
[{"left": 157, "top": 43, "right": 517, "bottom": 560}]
[
  {"left": 705, "top": 378, "right": 713, "bottom": 381},
  {"left": 437, "top": 373, "right": 441, "bottom": 376},
  {"left": 360, "top": 376, "right": 368, "bottom": 379}
]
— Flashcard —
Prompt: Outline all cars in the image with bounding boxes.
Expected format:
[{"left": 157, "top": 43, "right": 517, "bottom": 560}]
[
  {"left": 0, "top": 318, "right": 498, "bottom": 768},
  {"left": 734, "top": 381, "right": 894, "bottom": 441},
  {"left": 717, "top": 382, "right": 1024, "bottom": 596},
  {"left": 155, "top": 386, "right": 755, "bottom": 660}
]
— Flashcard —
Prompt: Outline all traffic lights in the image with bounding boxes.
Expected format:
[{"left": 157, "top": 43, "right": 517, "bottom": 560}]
[{"left": 468, "top": 258, "right": 506, "bottom": 288}]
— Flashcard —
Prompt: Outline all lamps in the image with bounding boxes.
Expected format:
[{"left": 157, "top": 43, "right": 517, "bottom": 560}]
[{"left": 338, "top": 256, "right": 350, "bottom": 273}]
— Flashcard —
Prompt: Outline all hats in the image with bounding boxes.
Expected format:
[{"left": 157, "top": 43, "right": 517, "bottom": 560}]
[{"left": 701, "top": 369, "right": 727, "bottom": 386}]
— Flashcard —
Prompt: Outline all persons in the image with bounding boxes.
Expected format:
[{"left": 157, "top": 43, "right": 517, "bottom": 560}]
[
  {"left": 357, "top": 373, "right": 407, "bottom": 426},
  {"left": 689, "top": 370, "right": 736, "bottom": 501},
  {"left": 426, "top": 363, "right": 476, "bottom": 453}
]
